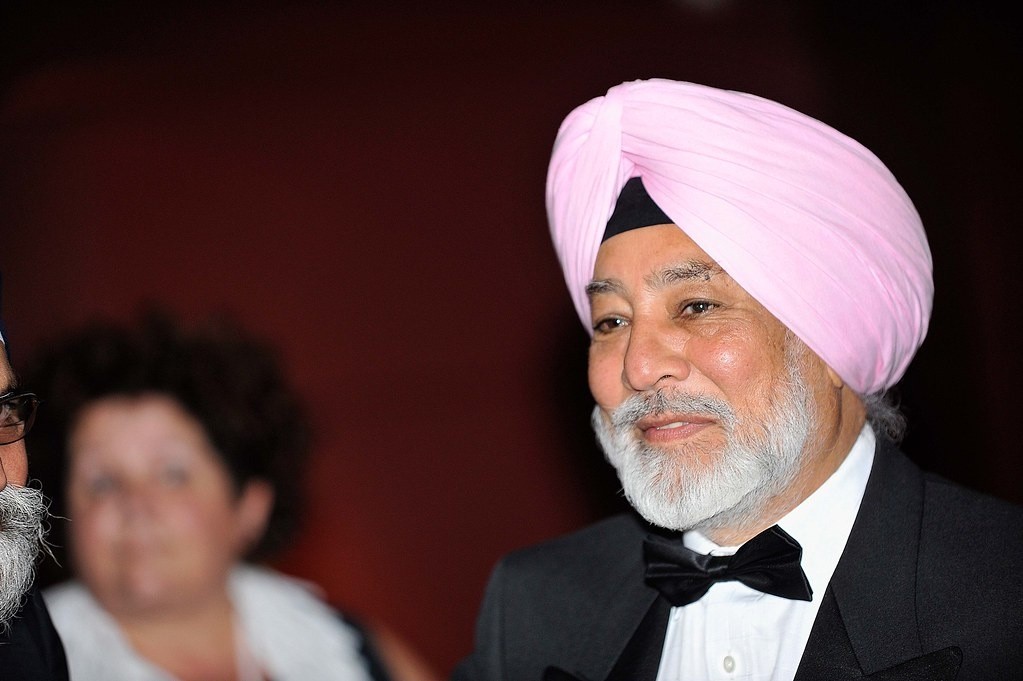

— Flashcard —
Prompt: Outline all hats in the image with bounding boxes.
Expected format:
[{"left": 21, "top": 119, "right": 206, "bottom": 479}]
[{"left": 543, "top": 75, "right": 942, "bottom": 392}]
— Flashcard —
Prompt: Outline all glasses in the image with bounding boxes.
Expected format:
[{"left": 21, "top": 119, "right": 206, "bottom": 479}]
[{"left": 1, "top": 391, "right": 41, "bottom": 448}]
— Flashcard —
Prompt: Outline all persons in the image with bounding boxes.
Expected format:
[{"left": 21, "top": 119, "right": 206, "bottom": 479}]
[
  {"left": 2, "top": 307, "right": 419, "bottom": 681},
  {"left": 0, "top": 336, "right": 55, "bottom": 666},
  {"left": 461, "top": 74, "right": 1023, "bottom": 681}
]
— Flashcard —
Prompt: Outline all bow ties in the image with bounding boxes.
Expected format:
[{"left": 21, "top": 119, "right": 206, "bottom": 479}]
[{"left": 646, "top": 523, "right": 812, "bottom": 608}]
